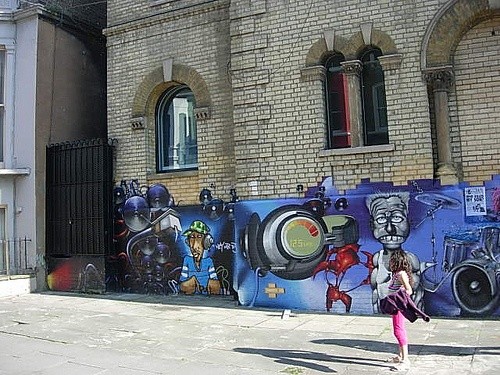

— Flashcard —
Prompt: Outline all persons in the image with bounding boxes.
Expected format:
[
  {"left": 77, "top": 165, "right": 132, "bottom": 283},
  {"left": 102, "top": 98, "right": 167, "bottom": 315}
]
[{"left": 386, "top": 249, "right": 413, "bottom": 372}]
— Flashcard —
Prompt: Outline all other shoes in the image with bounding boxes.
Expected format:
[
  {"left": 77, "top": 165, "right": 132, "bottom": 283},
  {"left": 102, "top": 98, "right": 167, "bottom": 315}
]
[{"left": 383, "top": 357, "right": 410, "bottom": 372}]
